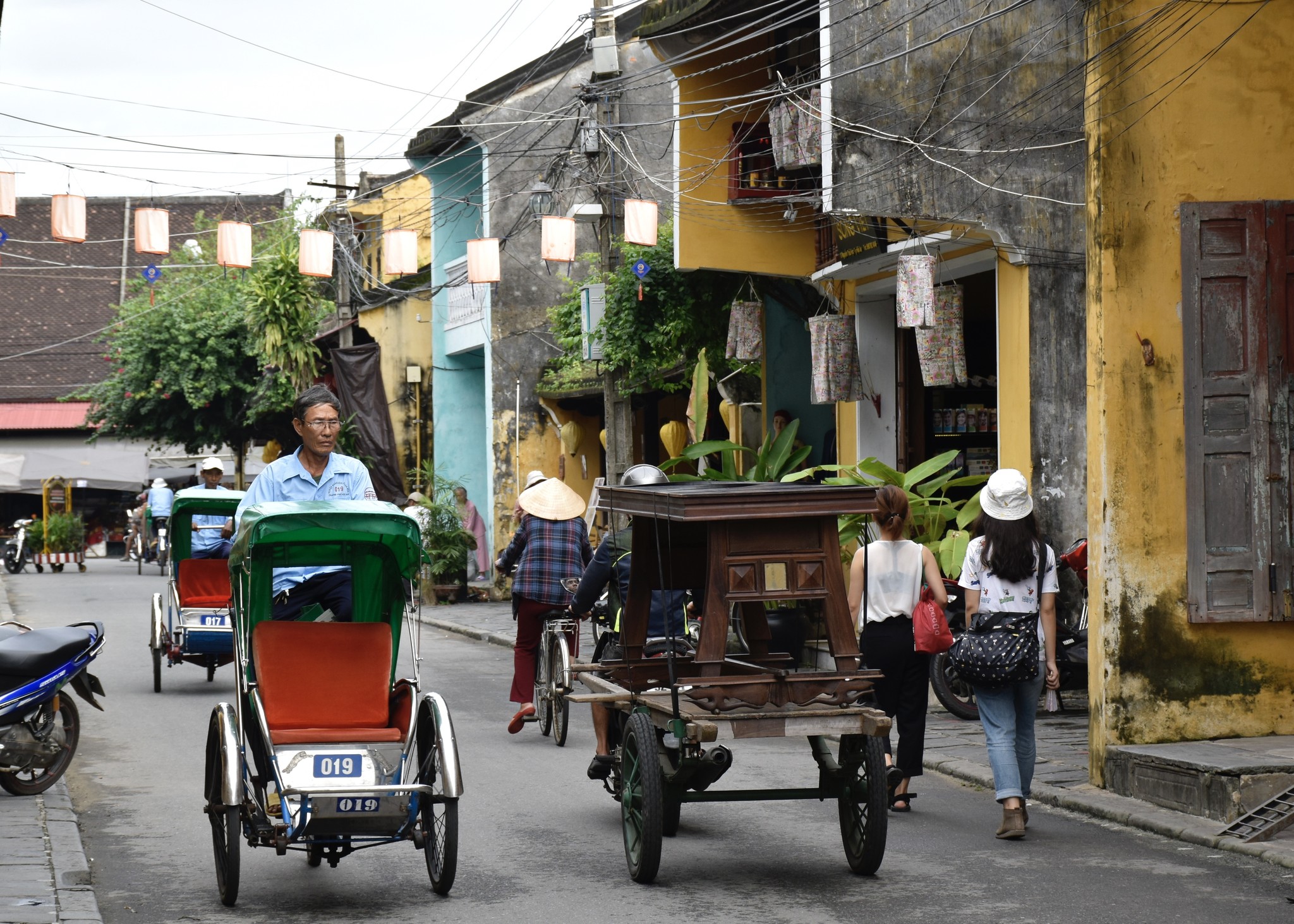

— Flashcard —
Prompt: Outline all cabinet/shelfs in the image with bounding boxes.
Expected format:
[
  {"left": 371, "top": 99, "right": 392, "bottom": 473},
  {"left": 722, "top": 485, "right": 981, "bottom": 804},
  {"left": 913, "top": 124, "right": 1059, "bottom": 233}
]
[{"left": 910, "top": 333, "right": 997, "bottom": 495}]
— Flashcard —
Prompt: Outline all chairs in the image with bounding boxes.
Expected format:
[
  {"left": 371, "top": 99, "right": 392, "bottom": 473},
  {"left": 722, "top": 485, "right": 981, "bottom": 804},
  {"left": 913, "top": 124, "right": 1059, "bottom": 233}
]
[
  {"left": 252, "top": 620, "right": 401, "bottom": 745},
  {"left": 179, "top": 559, "right": 233, "bottom": 608}
]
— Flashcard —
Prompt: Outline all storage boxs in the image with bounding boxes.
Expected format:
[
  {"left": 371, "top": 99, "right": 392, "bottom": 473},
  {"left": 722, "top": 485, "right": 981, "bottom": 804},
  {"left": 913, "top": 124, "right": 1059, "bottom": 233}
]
[{"left": 933, "top": 375, "right": 997, "bottom": 476}]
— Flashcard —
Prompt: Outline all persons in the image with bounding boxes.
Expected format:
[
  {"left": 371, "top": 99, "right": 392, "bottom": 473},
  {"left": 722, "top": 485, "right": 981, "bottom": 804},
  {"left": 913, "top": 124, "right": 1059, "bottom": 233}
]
[
  {"left": 757, "top": 404, "right": 836, "bottom": 483},
  {"left": 958, "top": 468, "right": 1059, "bottom": 838},
  {"left": 494, "top": 476, "right": 599, "bottom": 734},
  {"left": 119, "top": 457, "right": 241, "bottom": 584},
  {"left": 569, "top": 463, "right": 716, "bottom": 780},
  {"left": 848, "top": 485, "right": 948, "bottom": 811},
  {"left": 563, "top": 578, "right": 580, "bottom": 593},
  {"left": 403, "top": 471, "right": 548, "bottom": 582},
  {"left": 232, "top": 385, "right": 378, "bottom": 531}
]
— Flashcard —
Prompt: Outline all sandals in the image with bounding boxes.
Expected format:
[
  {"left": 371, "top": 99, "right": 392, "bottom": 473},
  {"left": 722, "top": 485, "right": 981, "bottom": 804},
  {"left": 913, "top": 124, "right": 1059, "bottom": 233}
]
[
  {"left": 265, "top": 792, "right": 282, "bottom": 816},
  {"left": 889, "top": 789, "right": 918, "bottom": 812},
  {"left": 886, "top": 764, "right": 903, "bottom": 793}
]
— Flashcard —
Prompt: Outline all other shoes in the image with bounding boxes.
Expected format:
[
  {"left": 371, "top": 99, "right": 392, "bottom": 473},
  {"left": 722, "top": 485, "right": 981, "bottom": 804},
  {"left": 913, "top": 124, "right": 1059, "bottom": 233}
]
[
  {"left": 1023, "top": 810, "right": 1029, "bottom": 829},
  {"left": 120, "top": 556, "right": 130, "bottom": 561},
  {"left": 587, "top": 756, "right": 611, "bottom": 779},
  {"left": 150, "top": 541, "right": 158, "bottom": 549},
  {"left": 996, "top": 807, "right": 1025, "bottom": 840}
]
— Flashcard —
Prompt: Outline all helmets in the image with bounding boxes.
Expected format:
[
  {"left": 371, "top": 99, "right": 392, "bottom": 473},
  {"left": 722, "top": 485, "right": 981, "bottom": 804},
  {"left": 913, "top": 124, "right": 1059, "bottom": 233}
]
[{"left": 620, "top": 463, "right": 669, "bottom": 484}]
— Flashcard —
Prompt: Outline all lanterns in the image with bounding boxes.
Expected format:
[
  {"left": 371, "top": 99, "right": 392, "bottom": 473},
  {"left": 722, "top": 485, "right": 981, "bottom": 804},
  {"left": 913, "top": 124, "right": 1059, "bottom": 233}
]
[{"left": 0, "top": 170, "right": 660, "bottom": 306}]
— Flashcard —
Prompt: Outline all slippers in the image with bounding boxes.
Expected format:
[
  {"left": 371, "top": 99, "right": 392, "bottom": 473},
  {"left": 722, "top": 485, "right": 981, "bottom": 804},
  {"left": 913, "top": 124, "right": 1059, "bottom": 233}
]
[
  {"left": 475, "top": 575, "right": 487, "bottom": 580},
  {"left": 507, "top": 706, "right": 535, "bottom": 734}
]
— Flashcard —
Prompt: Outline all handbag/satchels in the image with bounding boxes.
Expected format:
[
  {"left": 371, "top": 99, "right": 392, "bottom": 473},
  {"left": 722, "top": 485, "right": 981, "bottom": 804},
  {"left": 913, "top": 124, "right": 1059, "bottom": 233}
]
[
  {"left": 945, "top": 612, "right": 1039, "bottom": 690},
  {"left": 912, "top": 578, "right": 963, "bottom": 655}
]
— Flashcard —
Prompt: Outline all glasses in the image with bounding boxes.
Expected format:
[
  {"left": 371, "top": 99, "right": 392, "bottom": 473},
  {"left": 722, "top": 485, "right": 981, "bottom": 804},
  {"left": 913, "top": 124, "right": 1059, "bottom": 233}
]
[{"left": 301, "top": 419, "right": 345, "bottom": 431}]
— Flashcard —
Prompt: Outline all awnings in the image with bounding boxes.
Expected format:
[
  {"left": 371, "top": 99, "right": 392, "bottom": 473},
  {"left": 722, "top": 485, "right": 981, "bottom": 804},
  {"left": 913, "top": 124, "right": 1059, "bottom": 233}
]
[{"left": 0, "top": 447, "right": 270, "bottom": 496}]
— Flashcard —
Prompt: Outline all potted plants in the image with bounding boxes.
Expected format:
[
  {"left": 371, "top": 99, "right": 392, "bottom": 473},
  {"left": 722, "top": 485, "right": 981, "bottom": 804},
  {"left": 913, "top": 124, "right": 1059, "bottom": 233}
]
[{"left": 406, "top": 457, "right": 477, "bottom": 603}]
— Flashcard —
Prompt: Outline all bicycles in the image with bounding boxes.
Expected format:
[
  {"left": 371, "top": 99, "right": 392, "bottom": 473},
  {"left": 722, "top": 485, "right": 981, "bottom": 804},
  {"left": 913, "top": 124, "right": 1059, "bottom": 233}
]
[
  {"left": 128, "top": 509, "right": 169, "bottom": 575},
  {"left": 499, "top": 565, "right": 579, "bottom": 747}
]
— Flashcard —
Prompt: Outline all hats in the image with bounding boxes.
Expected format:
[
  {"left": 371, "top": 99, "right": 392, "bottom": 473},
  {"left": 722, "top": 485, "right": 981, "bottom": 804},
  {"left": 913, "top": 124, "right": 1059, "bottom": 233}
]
[
  {"left": 524, "top": 469, "right": 547, "bottom": 488},
  {"left": 405, "top": 492, "right": 424, "bottom": 504},
  {"left": 202, "top": 457, "right": 224, "bottom": 470},
  {"left": 518, "top": 477, "right": 586, "bottom": 520},
  {"left": 980, "top": 468, "right": 1033, "bottom": 520},
  {"left": 151, "top": 478, "right": 168, "bottom": 488}
]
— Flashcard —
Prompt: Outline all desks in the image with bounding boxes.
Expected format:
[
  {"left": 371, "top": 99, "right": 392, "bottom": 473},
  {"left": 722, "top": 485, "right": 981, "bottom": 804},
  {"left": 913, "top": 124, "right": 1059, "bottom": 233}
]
[{"left": 594, "top": 484, "right": 880, "bottom": 715}]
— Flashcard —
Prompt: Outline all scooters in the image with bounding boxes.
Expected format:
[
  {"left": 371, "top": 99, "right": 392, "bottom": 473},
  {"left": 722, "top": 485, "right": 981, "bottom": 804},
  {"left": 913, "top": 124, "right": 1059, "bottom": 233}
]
[
  {"left": 929, "top": 535, "right": 1088, "bottom": 720},
  {"left": 2, "top": 518, "right": 44, "bottom": 576},
  {"left": 0, "top": 620, "right": 107, "bottom": 792}
]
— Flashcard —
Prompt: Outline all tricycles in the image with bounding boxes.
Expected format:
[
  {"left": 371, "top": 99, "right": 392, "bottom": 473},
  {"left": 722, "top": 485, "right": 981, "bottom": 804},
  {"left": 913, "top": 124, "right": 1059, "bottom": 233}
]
[
  {"left": 150, "top": 490, "right": 250, "bottom": 692},
  {"left": 562, "top": 481, "right": 898, "bottom": 882},
  {"left": 203, "top": 502, "right": 464, "bottom": 908}
]
